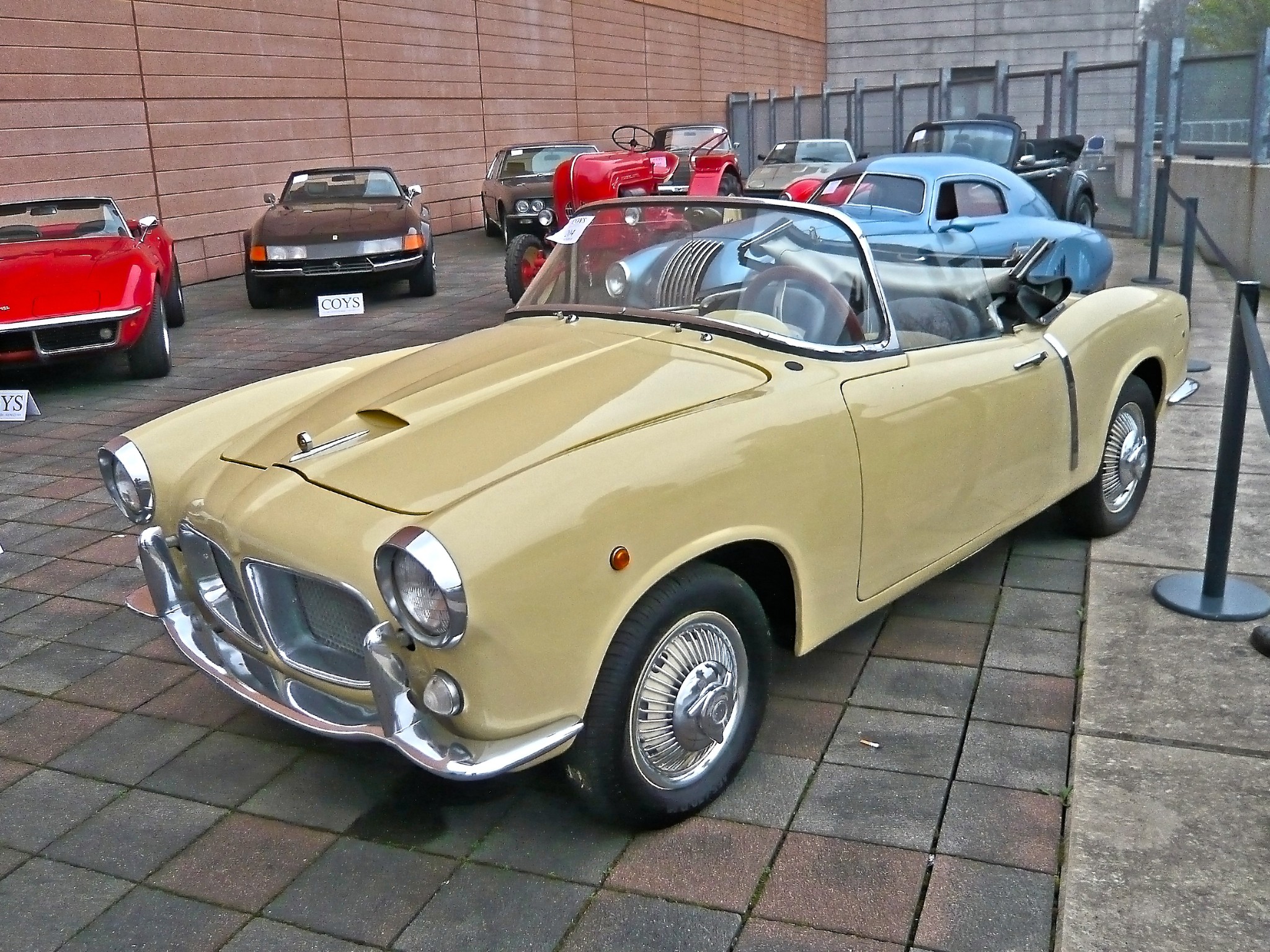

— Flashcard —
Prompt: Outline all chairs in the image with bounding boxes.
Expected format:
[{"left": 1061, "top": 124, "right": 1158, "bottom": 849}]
[
  {"left": 0, "top": 225, "right": 39, "bottom": 240},
  {"left": 718, "top": 287, "right": 824, "bottom": 342},
  {"left": 856, "top": 297, "right": 980, "bottom": 349},
  {"left": 951, "top": 134, "right": 971, "bottom": 155},
  {"left": 504, "top": 162, "right": 525, "bottom": 177},
  {"left": 305, "top": 182, "right": 329, "bottom": 198},
  {"left": 1079, "top": 136, "right": 1105, "bottom": 169},
  {"left": 646, "top": 151, "right": 680, "bottom": 183},
  {"left": 73, "top": 220, "right": 121, "bottom": 236}
]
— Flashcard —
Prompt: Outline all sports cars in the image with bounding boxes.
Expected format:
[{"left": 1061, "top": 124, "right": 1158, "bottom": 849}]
[
  {"left": 243, "top": 165, "right": 438, "bottom": 309},
  {"left": 480, "top": 143, "right": 600, "bottom": 251},
  {"left": 94, "top": 189, "right": 1200, "bottom": 834},
  {"left": 0, "top": 196, "right": 186, "bottom": 382},
  {"left": 480, "top": 114, "right": 1102, "bottom": 305}
]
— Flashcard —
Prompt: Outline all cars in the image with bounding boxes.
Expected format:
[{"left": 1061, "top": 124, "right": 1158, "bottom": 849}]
[{"left": 604, "top": 151, "right": 1114, "bottom": 312}]
